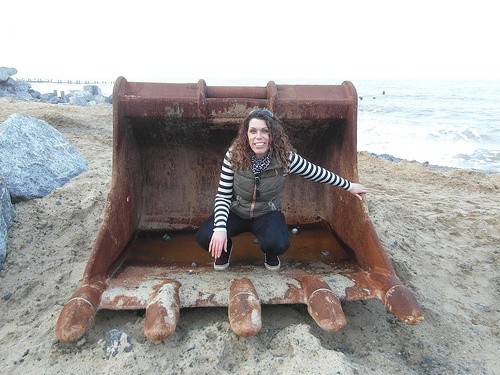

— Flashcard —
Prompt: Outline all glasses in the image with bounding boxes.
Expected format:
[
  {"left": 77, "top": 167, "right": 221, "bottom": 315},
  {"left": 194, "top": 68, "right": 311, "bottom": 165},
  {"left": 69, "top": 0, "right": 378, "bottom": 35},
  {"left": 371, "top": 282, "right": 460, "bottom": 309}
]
[{"left": 248, "top": 109, "right": 273, "bottom": 117}]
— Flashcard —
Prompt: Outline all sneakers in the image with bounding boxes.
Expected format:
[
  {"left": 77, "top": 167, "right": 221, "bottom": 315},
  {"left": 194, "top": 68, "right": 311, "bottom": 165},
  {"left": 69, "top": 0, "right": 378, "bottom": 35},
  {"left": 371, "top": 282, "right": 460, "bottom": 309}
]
[
  {"left": 214, "top": 239, "right": 233, "bottom": 271},
  {"left": 263, "top": 251, "right": 282, "bottom": 270}
]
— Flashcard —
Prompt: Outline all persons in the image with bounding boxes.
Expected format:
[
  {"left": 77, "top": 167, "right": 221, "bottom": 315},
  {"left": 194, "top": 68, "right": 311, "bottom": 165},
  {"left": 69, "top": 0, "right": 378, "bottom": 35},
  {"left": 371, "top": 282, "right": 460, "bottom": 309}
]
[{"left": 194, "top": 105, "right": 369, "bottom": 272}]
